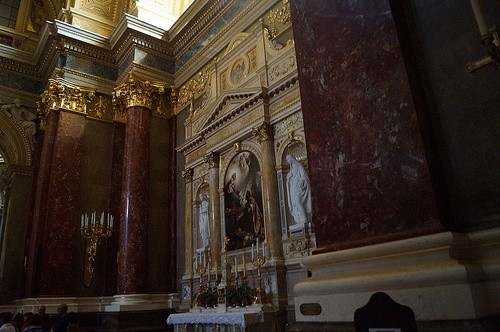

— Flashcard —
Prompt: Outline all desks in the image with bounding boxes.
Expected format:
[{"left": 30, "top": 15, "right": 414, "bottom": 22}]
[{"left": 166, "top": 303, "right": 276, "bottom": 332}]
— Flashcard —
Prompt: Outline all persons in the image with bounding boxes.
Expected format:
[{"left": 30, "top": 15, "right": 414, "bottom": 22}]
[
  {"left": 285, "top": 155, "right": 313, "bottom": 223},
  {"left": 2, "top": 98, "right": 37, "bottom": 151},
  {"left": 101, "top": 318, "right": 118, "bottom": 332},
  {"left": 0, "top": 303, "right": 81, "bottom": 332},
  {"left": 198, "top": 194, "right": 210, "bottom": 247}
]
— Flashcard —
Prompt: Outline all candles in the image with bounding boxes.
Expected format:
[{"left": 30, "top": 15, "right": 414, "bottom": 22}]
[
  {"left": 256, "top": 238, "right": 259, "bottom": 254},
  {"left": 100, "top": 211, "right": 104, "bottom": 225},
  {"left": 235, "top": 257, "right": 238, "bottom": 277},
  {"left": 92, "top": 211, "right": 96, "bottom": 224},
  {"left": 81, "top": 213, "right": 89, "bottom": 227},
  {"left": 107, "top": 213, "right": 114, "bottom": 227},
  {"left": 242, "top": 255, "right": 245, "bottom": 276},
  {"left": 215, "top": 260, "right": 218, "bottom": 280},
  {"left": 208, "top": 262, "right": 210, "bottom": 281},
  {"left": 196, "top": 257, "right": 198, "bottom": 268},
  {"left": 252, "top": 244, "right": 254, "bottom": 258},
  {"left": 200, "top": 250, "right": 202, "bottom": 264},
  {"left": 262, "top": 242, "right": 265, "bottom": 256},
  {"left": 204, "top": 255, "right": 206, "bottom": 266}
]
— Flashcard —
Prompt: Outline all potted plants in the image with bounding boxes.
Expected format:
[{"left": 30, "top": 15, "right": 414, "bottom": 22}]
[
  {"left": 194, "top": 290, "right": 218, "bottom": 307},
  {"left": 229, "top": 284, "right": 256, "bottom": 307}
]
